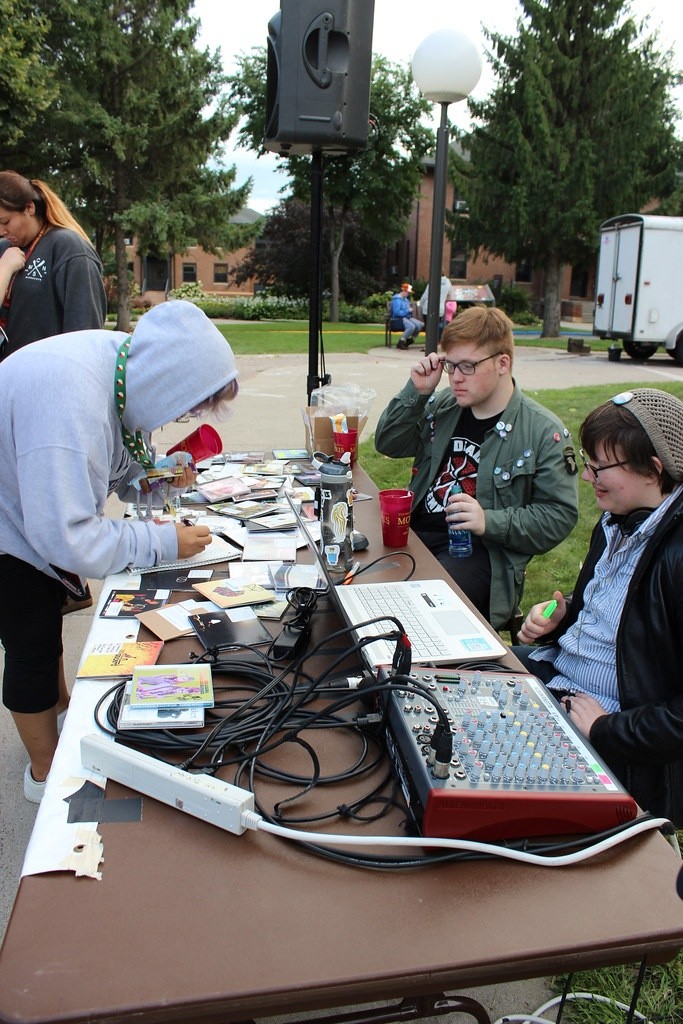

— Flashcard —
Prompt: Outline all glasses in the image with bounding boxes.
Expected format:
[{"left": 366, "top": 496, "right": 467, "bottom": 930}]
[
  {"left": 578, "top": 448, "right": 638, "bottom": 484},
  {"left": 439, "top": 351, "right": 512, "bottom": 376},
  {"left": 174, "top": 414, "right": 196, "bottom": 423}
]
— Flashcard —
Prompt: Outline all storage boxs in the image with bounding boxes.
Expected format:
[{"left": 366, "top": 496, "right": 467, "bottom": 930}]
[{"left": 303, "top": 406, "right": 368, "bottom": 460}]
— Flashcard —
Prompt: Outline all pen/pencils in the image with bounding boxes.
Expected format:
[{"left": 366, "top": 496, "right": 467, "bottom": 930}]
[{"left": 183, "top": 520, "right": 195, "bottom": 526}]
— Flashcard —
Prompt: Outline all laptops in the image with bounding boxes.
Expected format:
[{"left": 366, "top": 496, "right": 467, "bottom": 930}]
[{"left": 284, "top": 490, "right": 508, "bottom": 668}]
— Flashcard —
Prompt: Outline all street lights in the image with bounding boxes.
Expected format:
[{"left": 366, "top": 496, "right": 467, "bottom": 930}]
[{"left": 410, "top": 27, "right": 483, "bottom": 357}]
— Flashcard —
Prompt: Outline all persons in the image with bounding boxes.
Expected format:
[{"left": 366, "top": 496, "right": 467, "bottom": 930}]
[
  {"left": 0, "top": 300, "right": 242, "bottom": 806},
  {"left": 0, "top": 170, "right": 106, "bottom": 617},
  {"left": 509, "top": 388, "right": 683, "bottom": 837},
  {"left": 391, "top": 283, "right": 426, "bottom": 353},
  {"left": 374, "top": 303, "right": 578, "bottom": 630}
]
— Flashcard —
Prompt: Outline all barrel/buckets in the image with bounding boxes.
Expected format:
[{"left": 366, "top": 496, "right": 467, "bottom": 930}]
[{"left": 608, "top": 348, "right": 623, "bottom": 361}]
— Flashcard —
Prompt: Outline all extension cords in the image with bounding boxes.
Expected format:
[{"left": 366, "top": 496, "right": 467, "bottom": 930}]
[{"left": 80, "top": 733, "right": 254, "bottom": 835}]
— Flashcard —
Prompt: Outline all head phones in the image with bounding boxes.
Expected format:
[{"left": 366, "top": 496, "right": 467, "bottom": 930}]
[{"left": 607, "top": 507, "right": 656, "bottom": 536}]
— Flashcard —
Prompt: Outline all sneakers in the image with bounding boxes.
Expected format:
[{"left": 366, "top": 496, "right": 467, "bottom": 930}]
[
  {"left": 23, "top": 762, "right": 47, "bottom": 804},
  {"left": 57, "top": 695, "right": 71, "bottom": 737}
]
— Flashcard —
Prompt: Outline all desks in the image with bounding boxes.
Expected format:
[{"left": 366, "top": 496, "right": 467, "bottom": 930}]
[{"left": 1, "top": 449, "right": 683, "bottom": 1024}]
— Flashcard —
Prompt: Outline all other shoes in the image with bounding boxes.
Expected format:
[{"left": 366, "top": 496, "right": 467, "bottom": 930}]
[
  {"left": 61, "top": 594, "right": 93, "bottom": 616},
  {"left": 405, "top": 338, "right": 414, "bottom": 347},
  {"left": 396, "top": 339, "right": 408, "bottom": 350}
]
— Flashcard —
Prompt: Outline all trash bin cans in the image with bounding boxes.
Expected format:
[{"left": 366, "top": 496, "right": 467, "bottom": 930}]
[{"left": 608, "top": 348, "right": 623, "bottom": 361}]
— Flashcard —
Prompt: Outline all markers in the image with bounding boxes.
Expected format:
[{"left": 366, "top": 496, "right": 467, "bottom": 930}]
[
  {"left": 520, "top": 600, "right": 558, "bottom": 646},
  {"left": 343, "top": 561, "right": 360, "bottom": 585}
]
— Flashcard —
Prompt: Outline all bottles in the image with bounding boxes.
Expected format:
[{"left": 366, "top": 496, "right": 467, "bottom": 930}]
[
  {"left": 311, "top": 452, "right": 354, "bottom": 555},
  {"left": 447, "top": 485, "right": 472, "bottom": 558}
]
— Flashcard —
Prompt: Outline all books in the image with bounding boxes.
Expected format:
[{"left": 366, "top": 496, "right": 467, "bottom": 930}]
[{"left": 125, "top": 531, "right": 242, "bottom": 576}]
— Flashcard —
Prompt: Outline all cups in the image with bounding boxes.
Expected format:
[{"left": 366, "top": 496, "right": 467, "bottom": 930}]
[
  {"left": 333, "top": 429, "right": 357, "bottom": 469},
  {"left": 379, "top": 490, "right": 414, "bottom": 547},
  {"left": 165, "top": 424, "right": 223, "bottom": 464}
]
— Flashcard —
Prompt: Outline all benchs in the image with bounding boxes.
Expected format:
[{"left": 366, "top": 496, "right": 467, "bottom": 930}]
[{"left": 385, "top": 301, "right": 426, "bottom": 348}]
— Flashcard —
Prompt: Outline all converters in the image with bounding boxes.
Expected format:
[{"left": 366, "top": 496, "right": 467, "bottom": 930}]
[{"left": 273, "top": 622, "right": 306, "bottom": 658}]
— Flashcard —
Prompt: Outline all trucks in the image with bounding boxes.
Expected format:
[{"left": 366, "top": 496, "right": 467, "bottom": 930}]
[{"left": 591, "top": 212, "right": 683, "bottom": 367}]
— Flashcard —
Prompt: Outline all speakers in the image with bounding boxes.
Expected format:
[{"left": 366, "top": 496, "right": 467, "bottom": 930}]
[{"left": 265, "top": 0, "right": 375, "bottom": 156}]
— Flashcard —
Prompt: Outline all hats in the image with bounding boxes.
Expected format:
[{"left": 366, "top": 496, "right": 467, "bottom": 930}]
[
  {"left": 611, "top": 388, "right": 683, "bottom": 482},
  {"left": 401, "top": 283, "right": 415, "bottom": 293}
]
